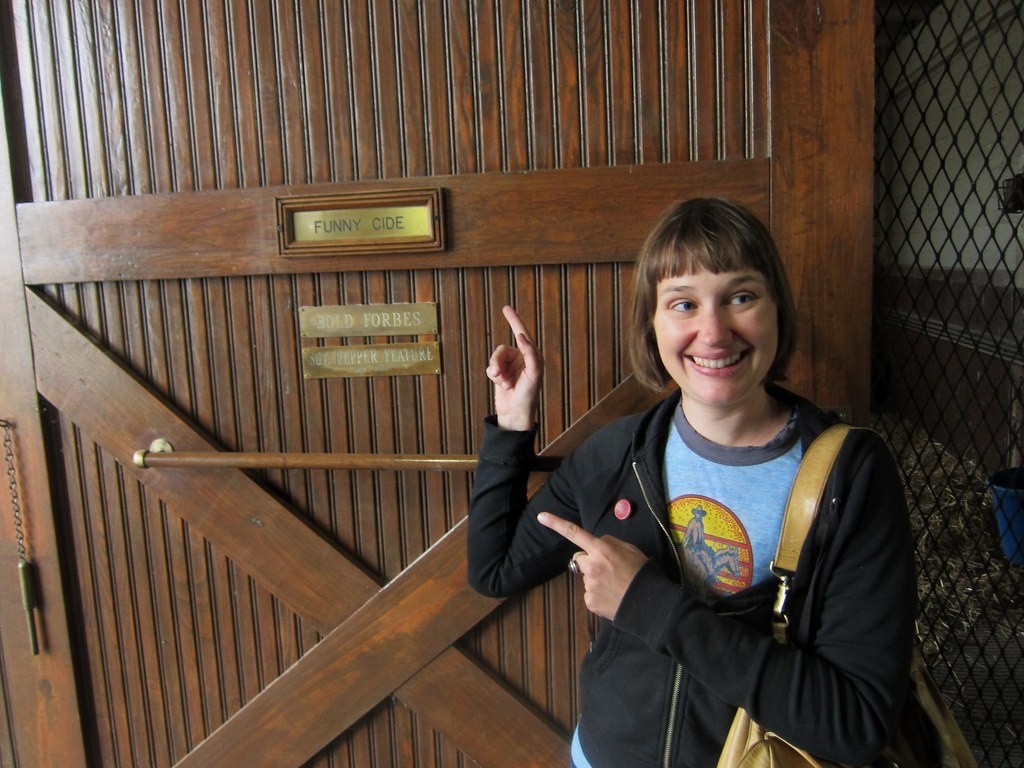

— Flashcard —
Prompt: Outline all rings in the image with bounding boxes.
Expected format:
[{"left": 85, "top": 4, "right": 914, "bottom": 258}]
[{"left": 568, "top": 552, "right": 586, "bottom": 576}]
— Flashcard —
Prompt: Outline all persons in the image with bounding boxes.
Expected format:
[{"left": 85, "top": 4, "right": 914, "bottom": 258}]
[{"left": 467, "top": 197, "right": 917, "bottom": 767}]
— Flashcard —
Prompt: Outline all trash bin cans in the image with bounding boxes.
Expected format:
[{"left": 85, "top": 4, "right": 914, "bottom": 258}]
[{"left": 987, "top": 468, "right": 1024, "bottom": 567}]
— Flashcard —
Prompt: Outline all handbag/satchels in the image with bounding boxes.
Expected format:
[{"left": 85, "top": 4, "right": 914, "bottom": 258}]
[{"left": 716, "top": 421, "right": 981, "bottom": 768}]
